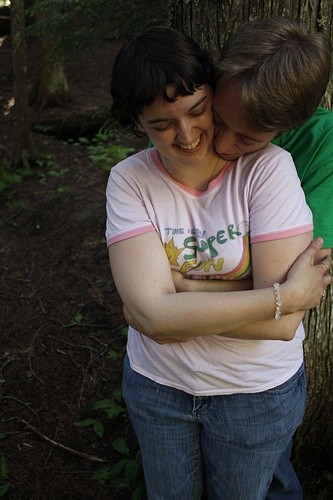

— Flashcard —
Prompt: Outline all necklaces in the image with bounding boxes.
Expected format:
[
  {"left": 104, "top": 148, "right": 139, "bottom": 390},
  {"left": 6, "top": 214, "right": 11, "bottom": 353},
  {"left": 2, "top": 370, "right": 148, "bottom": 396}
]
[{"left": 155, "top": 157, "right": 221, "bottom": 191}]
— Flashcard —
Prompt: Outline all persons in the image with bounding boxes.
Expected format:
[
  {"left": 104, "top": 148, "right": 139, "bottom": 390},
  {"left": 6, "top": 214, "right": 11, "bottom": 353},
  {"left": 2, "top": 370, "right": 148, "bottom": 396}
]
[
  {"left": 120, "top": 17, "right": 333, "bottom": 500},
  {"left": 103, "top": 26, "right": 333, "bottom": 500}
]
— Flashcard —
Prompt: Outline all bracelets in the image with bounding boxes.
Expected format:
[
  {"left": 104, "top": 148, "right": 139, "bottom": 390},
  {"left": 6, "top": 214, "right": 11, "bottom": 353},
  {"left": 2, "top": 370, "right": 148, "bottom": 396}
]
[{"left": 273, "top": 282, "right": 282, "bottom": 320}]
